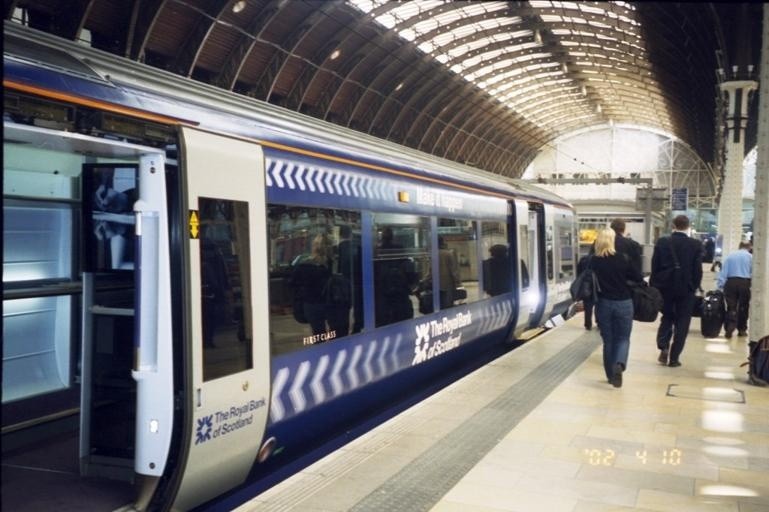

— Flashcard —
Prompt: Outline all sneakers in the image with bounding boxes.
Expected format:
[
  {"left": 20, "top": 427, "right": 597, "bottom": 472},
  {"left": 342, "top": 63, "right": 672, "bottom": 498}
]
[
  {"left": 658, "top": 354, "right": 682, "bottom": 367},
  {"left": 725, "top": 330, "right": 732, "bottom": 338},
  {"left": 607, "top": 364, "right": 622, "bottom": 387},
  {"left": 738, "top": 331, "right": 748, "bottom": 337}
]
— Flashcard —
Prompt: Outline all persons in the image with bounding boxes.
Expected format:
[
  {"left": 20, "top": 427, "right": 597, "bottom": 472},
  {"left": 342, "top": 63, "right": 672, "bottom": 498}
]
[
  {"left": 576, "top": 243, "right": 599, "bottom": 330},
  {"left": 200, "top": 218, "right": 235, "bottom": 350},
  {"left": 585, "top": 226, "right": 643, "bottom": 388},
  {"left": 481, "top": 244, "right": 513, "bottom": 298},
  {"left": 700, "top": 234, "right": 716, "bottom": 264},
  {"left": 589, "top": 218, "right": 643, "bottom": 273},
  {"left": 716, "top": 239, "right": 753, "bottom": 339},
  {"left": 648, "top": 214, "right": 703, "bottom": 367},
  {"left": 288, "top": 223, "right": 463, "bottom": 345}
]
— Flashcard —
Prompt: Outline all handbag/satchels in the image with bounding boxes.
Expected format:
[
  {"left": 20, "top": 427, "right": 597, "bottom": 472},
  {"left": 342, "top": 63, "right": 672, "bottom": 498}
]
[
  {"left": 570, "top": 268, "right": 601, "bottom": 302},
  {"left": 625, "top": 279, "right": 665, "bottom": 322}
]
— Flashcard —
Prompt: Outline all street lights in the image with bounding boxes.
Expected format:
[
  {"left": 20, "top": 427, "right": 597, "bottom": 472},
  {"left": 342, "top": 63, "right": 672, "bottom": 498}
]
[{"left": 711, "top": 62, "right": 761, "bottom": 266}]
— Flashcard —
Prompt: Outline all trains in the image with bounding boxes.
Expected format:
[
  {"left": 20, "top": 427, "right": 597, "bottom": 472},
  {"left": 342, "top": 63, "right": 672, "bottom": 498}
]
[{"left": 2, "top": 21, "right": 581, "bottom": 512}]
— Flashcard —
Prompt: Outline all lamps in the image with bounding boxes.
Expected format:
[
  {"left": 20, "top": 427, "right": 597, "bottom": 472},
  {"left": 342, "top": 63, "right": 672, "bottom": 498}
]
[
  {"left": 229, "top": 2, "right": 248, "bottom": 15},
  {"left": 533, "top": 30, "right": 617, "bottom": 140}
]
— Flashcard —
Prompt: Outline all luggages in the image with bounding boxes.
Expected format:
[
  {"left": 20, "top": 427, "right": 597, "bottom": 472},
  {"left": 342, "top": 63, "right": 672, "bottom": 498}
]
[
  {"left": 700, "top": 289, "right": 725, "bottom": 338},
  {"left": 693, "top": 296, "right": 703, "bottom": 317}
]
